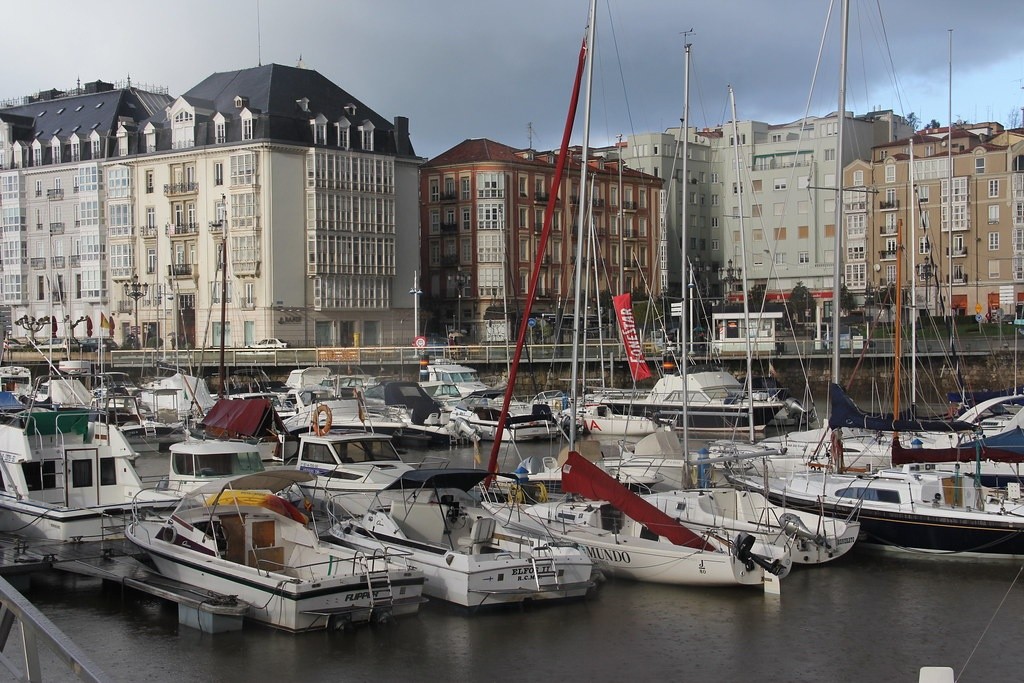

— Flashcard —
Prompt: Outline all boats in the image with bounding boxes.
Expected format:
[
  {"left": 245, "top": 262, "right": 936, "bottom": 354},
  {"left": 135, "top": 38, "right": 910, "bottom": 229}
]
[{"left": 0, "top": 196, "right": 596, "bottom": 633}]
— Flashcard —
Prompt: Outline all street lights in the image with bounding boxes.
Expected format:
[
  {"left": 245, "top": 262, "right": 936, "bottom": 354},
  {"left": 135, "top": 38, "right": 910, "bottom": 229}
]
[
  {"left": 447, "top": 264, "right": 473, "bottom": 345},
  {"left": 124, "top": 272, "right": 148, "bottom": 351},
  {"left": 408, "top": 270, "right": 423, "bottom": 359}
]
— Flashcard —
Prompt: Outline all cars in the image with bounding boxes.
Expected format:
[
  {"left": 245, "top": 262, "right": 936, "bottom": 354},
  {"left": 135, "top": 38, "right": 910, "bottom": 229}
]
[
  {"left": 244, "top": 336, "right": 291, "bottom": 349},
  {"left": 2, "top": 337, "right": 119, "bottom": 353}
]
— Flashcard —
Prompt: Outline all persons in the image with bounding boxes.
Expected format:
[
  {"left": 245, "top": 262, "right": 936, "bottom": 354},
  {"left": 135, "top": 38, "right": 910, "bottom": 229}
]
[
  {"left": 448, "top": 336, "right": 461, "bottom": 359},
  {"left": 915, "top": 330, "right": 920, "bottom": 352},
  {"left": 697, "top": 333, "right": 706, "bottom": 357},
  {"left": 147, "top": 329, "right": 153, "bottom": 343},
  {"left": 169, "top": 333, "right": 186, "bottom": 350}
]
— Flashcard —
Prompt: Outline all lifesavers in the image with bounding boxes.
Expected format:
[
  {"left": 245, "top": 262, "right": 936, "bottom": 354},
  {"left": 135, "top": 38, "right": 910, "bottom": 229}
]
[{"left": 312, "top": 406, "right": 334, "bottom": 437}]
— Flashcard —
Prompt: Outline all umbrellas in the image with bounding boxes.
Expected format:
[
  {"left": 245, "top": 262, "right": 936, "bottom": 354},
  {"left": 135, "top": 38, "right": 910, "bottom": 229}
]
[
  {"left": 168, "top": 331, "right": 177, "bottom": 339},
  {"left": 691, "top": 326, "right": 707, "bottom": 333}
]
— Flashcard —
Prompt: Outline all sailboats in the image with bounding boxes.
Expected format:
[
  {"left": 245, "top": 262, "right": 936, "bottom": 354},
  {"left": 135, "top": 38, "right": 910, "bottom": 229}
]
[{"left": 485, "top": 0, "right": 1024, "bottom": 594}]
